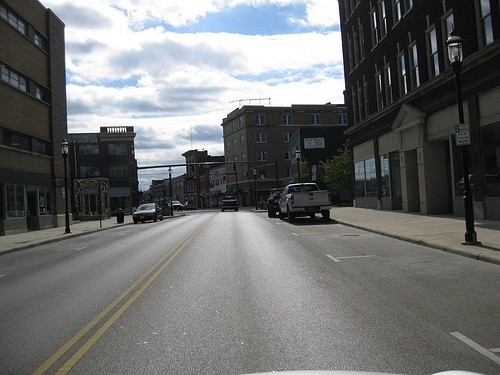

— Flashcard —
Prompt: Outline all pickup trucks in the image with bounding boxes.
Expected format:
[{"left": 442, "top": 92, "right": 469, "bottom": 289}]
[
  {"left": 221, "top": 195, "right": 240, "bottom": 212},
  {"left": 276, "top": 182, "right": 332, "bottom": 222}
]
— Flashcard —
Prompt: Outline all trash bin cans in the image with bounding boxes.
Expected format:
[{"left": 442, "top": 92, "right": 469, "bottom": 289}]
[{"left": 117, "top": 208, "right": 124, "bottom": 223}]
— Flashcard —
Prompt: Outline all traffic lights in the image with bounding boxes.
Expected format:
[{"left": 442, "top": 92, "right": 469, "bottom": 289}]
[
  {"left": 260, "top": 167, "right": 264, "bottom": 174},
  {"left": 193, "top": 175, "right": 197, "bottom": 182}
]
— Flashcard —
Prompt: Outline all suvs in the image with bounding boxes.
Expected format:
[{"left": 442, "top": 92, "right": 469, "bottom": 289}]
[{"left": 265, "top": 187, "right": 287, "bottom": 218}]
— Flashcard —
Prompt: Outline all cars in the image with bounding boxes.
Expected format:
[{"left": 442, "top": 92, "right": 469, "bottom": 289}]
[
  {"left": 184, "top": 200, "right": 197, "bottom": 211},
  {"left": 167, "top": 200, "right": 183, "bottom": 212},
  {"left": 131, "top": 202, "right": 164, "bottom": 223}
]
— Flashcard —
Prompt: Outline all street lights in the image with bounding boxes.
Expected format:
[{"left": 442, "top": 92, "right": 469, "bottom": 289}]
[
  {"left": 253, "top": 169, "right": 259, "bottom": 211},
  {"left": 446, "top": 24, "right": 481, "bottom": 245},
  {"left": 168, "top": 167, "right": 174, "bottom": 217},
  {"left": 60, "top": 139, "right": 73, "bottom": 234},
  {"left": 295, "top": 148, "right": 301, "bottom": 183}
]
